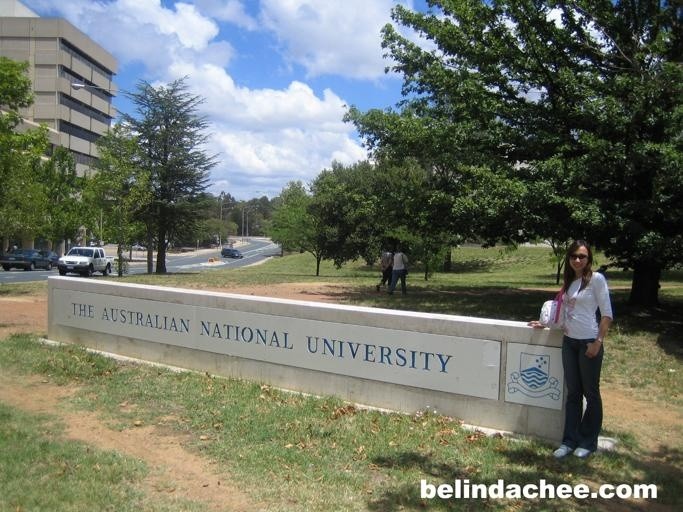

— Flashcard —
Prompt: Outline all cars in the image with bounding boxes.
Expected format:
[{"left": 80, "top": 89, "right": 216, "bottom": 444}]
[
  {"left": 0, "top": 249, "right": 52, "bottom": 271},
  {"left": 38, "top": 249, "right": 61, "bottom": 267},
  {"left": 131, "top": 244, "right": 146, "bottom": 251}
]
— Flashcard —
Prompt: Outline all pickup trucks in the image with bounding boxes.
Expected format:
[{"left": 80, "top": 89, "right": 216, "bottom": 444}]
[{"left": 57, "top": 247, "right": 114, "bottom": 276}]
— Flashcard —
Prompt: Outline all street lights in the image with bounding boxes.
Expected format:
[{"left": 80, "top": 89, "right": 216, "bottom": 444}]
[
  {"left": 218, "top": 203, "right": 233, "bottom": 249},
  {"left": 69, "top": 83, "right": 152, "bottom": 275},
  {"left": 240, "top": 205, "right": 259, "bottom": 242},
  {"left": 246, "top": 209, "right": 255, "bottom": 238},
  {"left": 221, "top": 207, "right": 233, "bottom": 211}
]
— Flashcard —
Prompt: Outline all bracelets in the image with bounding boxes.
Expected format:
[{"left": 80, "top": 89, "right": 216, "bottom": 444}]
[{"left": 596, "top": 336, "right": 604, "bottom": 342}]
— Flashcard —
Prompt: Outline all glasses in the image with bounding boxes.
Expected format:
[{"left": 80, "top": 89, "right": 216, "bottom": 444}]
[{"left": 571, "top": 254, "right": 588, "bottom": 260}]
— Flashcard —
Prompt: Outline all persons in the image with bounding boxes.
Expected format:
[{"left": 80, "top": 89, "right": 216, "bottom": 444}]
[
  {"left": 373, "top": 245, "right": 393, "bottom": 292},
  {"left": 387, "top": 245, "right": 409, "bottom": 298},
  {"left": 528, "top": 241, "right": 616, "bottom": 462}
]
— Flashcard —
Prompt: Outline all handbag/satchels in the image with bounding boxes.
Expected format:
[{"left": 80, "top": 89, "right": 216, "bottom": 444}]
[
  {"left": 387, "top": 266, "right": 392, "bottom": 277},
  {"left": 539, "top": 300, "right": 564, "bottom": 327},
  {"left": 405, "top": 269, "right": 408, "bottom": 275}
]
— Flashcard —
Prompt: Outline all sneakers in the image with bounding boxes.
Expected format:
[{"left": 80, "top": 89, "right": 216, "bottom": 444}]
[
  {"left": 572, "top": 447, "right": 590, "bottom": 457},
  {"left": 376, "top": 284, "right": 380, "bottom": 291},
  {"left": 553, "top": 444, "right": 573, "bottom": 457}
]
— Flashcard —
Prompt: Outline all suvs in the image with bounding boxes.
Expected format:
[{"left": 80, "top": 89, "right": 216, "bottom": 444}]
[{"left": 221, "top": 248, "right": 243, "bottom": 259}]
